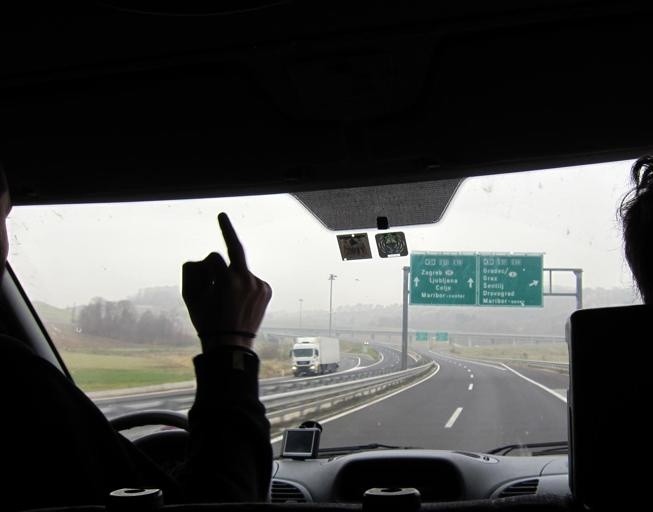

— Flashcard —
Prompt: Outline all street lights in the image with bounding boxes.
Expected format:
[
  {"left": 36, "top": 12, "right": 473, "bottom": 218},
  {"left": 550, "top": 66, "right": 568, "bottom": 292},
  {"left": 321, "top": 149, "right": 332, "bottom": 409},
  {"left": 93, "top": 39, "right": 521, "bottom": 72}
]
[{"left": 327, "top": 273, "right": 337, "bottom": 333}]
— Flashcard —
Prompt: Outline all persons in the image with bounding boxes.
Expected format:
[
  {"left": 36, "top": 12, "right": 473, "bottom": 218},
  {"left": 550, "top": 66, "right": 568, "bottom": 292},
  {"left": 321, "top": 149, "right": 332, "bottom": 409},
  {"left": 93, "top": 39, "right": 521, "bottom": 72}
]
[{"left": 0, "top": 153, "right": 274, "bottom": 511}]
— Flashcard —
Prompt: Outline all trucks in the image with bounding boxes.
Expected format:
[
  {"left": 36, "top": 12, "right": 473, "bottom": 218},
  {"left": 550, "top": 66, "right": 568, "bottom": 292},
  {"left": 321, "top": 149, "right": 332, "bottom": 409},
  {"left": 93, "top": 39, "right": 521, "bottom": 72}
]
[{"left": 287, "top": 336, "right": 340, "bottom": 377}]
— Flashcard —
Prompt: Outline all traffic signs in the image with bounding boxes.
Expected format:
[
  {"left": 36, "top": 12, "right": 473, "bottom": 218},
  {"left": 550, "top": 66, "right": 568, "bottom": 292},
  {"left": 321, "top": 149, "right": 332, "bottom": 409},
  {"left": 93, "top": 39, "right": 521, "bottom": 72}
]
[
  {"left": 407, "top": 251, "right": 476, "bottom": 307},
  {"left": 415, "top": 332, "right": 427, "bottom": 340},
  {"left": 478, "top": 252, "right": 542, "bottom": 307},
  {"left": 436, "top": 331, "right": 449, "bottom": 342}
]
